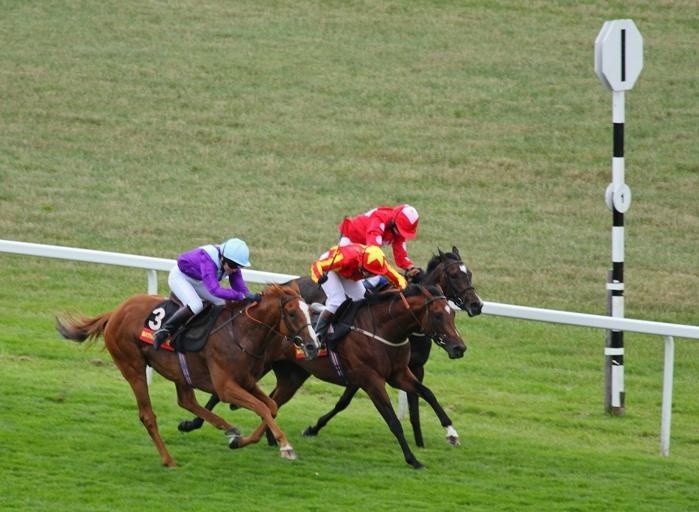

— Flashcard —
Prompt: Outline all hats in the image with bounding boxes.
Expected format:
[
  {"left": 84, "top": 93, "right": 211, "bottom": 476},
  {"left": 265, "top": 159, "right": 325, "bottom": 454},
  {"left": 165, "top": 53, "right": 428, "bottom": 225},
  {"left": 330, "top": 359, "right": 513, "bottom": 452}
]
[
  {"left": 390, "top": 204, "right": 420, "bottom": 240},
  {"left": 362, "top": 245, "right": 391, "bottom": 275}
]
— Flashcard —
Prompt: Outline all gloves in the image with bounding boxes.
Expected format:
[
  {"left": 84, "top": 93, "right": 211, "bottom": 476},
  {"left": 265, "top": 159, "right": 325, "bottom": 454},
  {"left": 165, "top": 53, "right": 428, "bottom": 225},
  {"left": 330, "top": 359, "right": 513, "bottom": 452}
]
[
  {"left": 245, "top": 291, "right": 261, "bottom": 303},
  {"left": 317, "top": 271, "right": 328, "bottom": 285},
  {"left": 404, "top": 266, "right": 425, "bottom": 284}
]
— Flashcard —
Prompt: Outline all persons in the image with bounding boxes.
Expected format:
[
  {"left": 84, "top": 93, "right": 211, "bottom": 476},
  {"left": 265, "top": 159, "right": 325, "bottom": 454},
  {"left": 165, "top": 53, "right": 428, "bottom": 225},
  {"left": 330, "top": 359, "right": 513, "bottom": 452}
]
[
  {"left": 338, "top": 205, "right": 420, "bottom": 278},
  {"left": 152, "top": 238, "right": 251, "bottom": 351},
  {"left": 310, "top": 243, "right": 407, "bottom": 350}
]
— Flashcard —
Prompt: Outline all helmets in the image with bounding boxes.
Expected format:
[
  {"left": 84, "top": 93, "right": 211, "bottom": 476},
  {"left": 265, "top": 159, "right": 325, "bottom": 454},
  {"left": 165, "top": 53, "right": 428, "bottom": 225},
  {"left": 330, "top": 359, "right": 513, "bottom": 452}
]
[{"left": 220, "top": 239, "right": 251, "bottom": 267}]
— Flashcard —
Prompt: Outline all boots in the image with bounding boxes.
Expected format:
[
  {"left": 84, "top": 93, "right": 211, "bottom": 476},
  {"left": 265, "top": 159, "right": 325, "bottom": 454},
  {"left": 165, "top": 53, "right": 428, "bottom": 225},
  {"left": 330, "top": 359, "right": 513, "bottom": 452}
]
[
  {"left": 154, "top": 304, "right": 195, "bottom": 351},
  {"left": 314, "top": 309, "right": 335, "bottom": 349}
]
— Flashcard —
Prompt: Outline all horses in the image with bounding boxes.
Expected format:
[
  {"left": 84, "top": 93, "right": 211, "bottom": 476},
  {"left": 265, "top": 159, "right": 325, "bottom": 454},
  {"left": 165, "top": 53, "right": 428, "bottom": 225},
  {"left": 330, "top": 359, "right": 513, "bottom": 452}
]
[
  {"left": 54, "top": 279, "right": 320, "bottom": 468},
  {"left": 229, "top": 246, "right": 484, "bottom": 448},
  {"left": 178, "top": 262, "right": 467, "bottom": 470}
]
